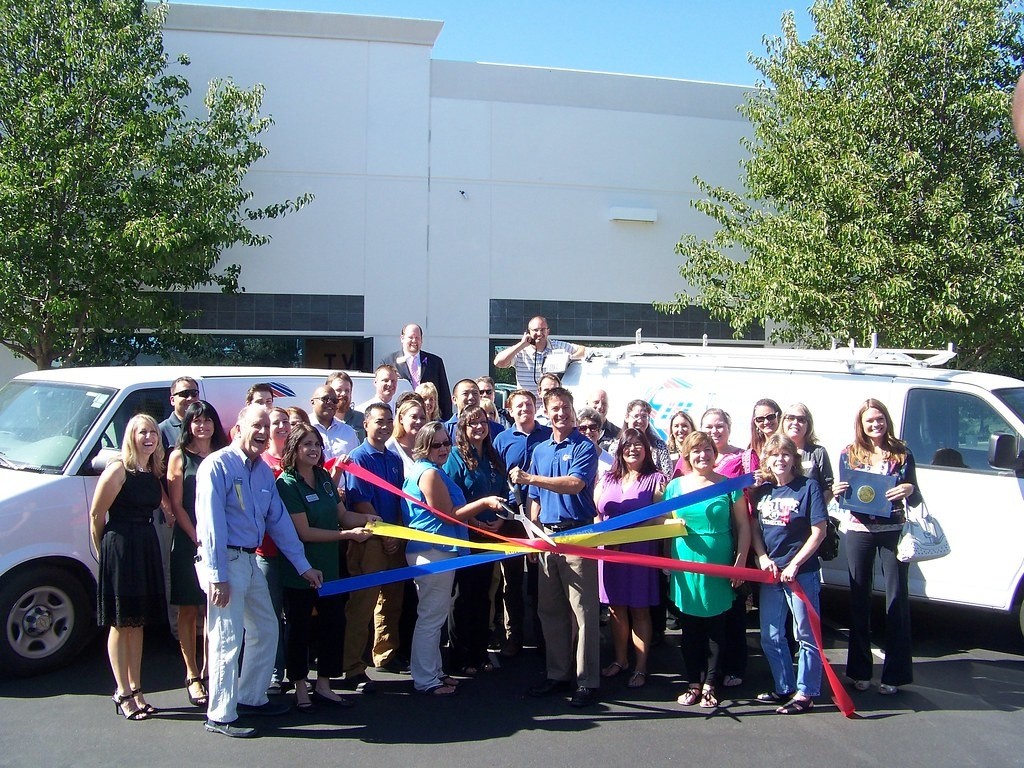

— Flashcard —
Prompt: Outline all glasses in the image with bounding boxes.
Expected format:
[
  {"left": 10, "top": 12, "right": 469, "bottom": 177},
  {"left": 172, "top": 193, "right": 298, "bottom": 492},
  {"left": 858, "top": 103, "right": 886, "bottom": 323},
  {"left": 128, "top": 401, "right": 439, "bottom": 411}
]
[
  {"left": 754, "top": 414, "right": 776, "bottom": 422},
  {"left": 480, "top": 390, "right": 493, "bottom": 394},
  {"left": 529, "top": 328, "right": 547, "bottom": 332},
  {"left": 578, "top": 424, "right": 600, "bottom": 433},
  {"left": 313, "top": 396, "right": 339, "bottom": 404},
  {"left": 173, "top": 390, "right": 200, "bottom": 398},
  {"left": 431, "top": 441, "right": 451, "bottom": 448},
  {"left": 784, "top": 415, "right": 807, "bottom": 423},
  {"left": 465, "top": 420, "right": 487, "bottom": 428}
]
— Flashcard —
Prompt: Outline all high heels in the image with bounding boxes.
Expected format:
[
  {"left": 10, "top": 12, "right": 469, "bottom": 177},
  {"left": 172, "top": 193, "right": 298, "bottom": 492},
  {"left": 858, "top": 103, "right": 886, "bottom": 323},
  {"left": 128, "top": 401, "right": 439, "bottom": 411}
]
[
  {"left": 200, "top": 677, "right": 208, "bottom": 695},
  {"left": 132, "top": 688, "right": 158, "bottom": 714},
  {"left": 112, "top": 689, "right": 149, "bottom": 721},
  {"left": 185, "top": 677, "right": 208, "bottom": 705}
]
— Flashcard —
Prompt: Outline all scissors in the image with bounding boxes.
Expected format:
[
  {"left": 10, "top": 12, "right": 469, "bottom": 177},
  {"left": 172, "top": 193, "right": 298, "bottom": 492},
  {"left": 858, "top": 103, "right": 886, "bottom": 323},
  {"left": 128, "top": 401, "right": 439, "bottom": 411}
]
[{"left": 493, "top": 473, "right": 557, "bottom": 566}]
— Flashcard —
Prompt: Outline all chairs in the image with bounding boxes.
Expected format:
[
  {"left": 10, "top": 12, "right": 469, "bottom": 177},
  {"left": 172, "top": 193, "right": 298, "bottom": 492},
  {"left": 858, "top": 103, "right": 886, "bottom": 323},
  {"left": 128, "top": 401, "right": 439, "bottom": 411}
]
[
  {"left": 96, "top": 401, "right": 127, "bottom": 449},
  {"left": 918, "top": 397, "right": 981, "bottom": 468}
]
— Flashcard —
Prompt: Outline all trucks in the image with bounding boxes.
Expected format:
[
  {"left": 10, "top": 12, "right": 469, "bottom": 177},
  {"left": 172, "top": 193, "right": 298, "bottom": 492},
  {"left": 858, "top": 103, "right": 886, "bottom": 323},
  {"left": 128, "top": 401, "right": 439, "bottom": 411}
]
[
  {"left": 557, "top": 341, "right": 1024, "bottom": 637},
  {"left": 0, "top": 363, "right": 418, "bottom": 684}
]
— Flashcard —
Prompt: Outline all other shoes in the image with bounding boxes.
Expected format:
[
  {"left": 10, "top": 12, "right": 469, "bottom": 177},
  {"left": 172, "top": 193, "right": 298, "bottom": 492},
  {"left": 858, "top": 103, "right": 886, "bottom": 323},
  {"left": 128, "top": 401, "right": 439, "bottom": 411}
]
[
  {"left": 204, "top": 720, "right": 256, "bottom": 738},
  {"left": 291, "top": 638, "right": 897, "bottom": 714},
  {"left": 236, "top": 698, "right": 290, "bottom": 715},
  {"left": 268, "top": 681, "right": 282, "bottom": 694}
]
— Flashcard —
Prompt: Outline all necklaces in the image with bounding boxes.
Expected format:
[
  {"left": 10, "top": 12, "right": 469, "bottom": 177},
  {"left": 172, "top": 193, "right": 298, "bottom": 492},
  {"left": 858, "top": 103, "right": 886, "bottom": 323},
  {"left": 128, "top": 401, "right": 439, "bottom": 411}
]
[{"left": 136, "top": 461, "right": 153, "bottom": 473}]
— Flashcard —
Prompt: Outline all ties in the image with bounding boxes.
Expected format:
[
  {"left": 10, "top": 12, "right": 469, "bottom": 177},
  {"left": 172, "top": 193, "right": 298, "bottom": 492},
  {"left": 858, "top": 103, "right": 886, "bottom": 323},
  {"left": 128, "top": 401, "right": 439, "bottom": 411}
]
[{"left": 410, "top": 356, "right": 420, "bottom": 391}]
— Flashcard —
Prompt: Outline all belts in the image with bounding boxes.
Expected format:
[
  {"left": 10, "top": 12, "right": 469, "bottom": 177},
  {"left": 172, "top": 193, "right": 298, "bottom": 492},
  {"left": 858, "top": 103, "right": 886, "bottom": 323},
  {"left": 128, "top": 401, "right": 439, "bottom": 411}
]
[
  {"left": 543, "top": 522, "right": 592, "bottom": 532},
  {"left": 227, "top": 545, "right": 257, "bottom": 554}
]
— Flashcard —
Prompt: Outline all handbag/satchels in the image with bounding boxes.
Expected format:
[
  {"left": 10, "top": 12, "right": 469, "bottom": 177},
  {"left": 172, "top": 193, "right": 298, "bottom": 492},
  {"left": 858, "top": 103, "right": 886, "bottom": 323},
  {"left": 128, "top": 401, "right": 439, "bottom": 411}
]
[
  {"left": 896, "top": 500, "right": 951, "bottom": 562},
  {"left": 816, "top": 524, "right": 840, "bottom": 561}
]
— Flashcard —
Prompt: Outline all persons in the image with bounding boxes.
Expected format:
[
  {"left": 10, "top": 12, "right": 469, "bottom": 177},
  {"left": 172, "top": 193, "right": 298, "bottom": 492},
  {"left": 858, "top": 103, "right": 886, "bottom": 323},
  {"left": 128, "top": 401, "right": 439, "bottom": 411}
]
[
  {"left": 493, "top": 316, "right": 586, "bottom": 413},
  {"left": 378, "top": 323, "right": 453, "bottom": 422},
  {"left": 831, "top": 397, "right": 922, "bottom": 695},
  {"left": 158, "top": 365, "right": 835, "bottom": 714},
  {"left": 89, "top": 414, "right": 167, "bottom": 720}
]
